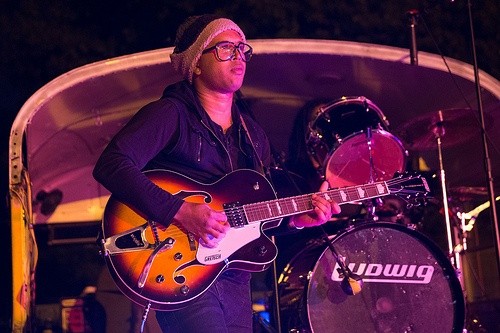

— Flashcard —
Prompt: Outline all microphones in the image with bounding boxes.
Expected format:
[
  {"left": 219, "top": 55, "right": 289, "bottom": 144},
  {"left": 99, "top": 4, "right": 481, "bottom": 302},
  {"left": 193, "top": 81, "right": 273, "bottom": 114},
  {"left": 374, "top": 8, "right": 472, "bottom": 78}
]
[
  {"left": 340, "top": 264, "right": 363, "bottom": 295},
  {"left": 405, "top": 9, "right": 418, "bottom": 66}
]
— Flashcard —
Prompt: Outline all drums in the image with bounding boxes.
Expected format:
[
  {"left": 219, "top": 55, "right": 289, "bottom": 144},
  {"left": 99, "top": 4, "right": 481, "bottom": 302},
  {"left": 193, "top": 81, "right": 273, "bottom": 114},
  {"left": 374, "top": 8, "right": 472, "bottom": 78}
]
[
  {"left": 301, "top": 93, "right": 407, "bottom": 205},
  {"left": 274, "top": 220, "right": 469, "bottom": 333}
]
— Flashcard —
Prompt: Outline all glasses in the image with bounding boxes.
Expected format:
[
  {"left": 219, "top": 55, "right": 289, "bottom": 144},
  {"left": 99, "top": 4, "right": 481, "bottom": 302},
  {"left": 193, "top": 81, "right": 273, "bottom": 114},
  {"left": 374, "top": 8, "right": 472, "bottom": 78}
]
[{"left": 199, "top": 41, "right": 253, "bottom": 62}]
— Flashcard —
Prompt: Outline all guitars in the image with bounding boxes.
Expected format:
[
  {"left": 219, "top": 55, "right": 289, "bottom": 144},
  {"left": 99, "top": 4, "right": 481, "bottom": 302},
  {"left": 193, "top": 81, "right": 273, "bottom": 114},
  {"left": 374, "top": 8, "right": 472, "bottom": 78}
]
[{"left": 100, "top": 166, "right": 432, "bottom": 312}]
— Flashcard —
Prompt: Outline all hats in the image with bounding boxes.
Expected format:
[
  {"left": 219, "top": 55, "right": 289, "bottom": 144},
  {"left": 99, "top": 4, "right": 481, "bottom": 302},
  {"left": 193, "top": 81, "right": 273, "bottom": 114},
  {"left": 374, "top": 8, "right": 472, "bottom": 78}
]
[{"left": 169, "top": 14, "right": 248, "bottom": 85}]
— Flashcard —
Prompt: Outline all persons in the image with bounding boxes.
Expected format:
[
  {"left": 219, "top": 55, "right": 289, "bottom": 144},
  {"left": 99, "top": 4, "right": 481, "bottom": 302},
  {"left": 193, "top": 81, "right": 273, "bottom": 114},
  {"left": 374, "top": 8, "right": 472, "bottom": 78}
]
[{"left": 92, "top": 15, "right": 341, "bottom": 333}]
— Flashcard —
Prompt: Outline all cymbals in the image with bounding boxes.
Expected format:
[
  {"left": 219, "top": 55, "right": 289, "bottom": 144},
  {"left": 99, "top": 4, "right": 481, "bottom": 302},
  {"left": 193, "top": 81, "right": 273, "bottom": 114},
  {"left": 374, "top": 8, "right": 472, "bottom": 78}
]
[{"left": 395, "top": 104, "right": 479, "bottom": 136}]
